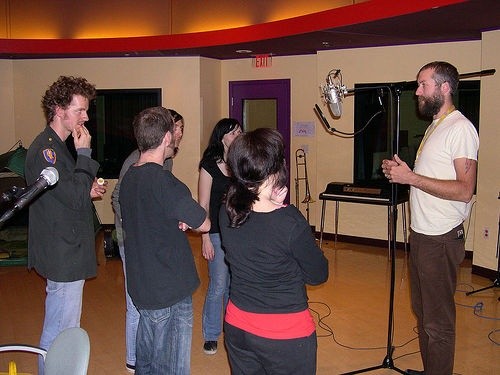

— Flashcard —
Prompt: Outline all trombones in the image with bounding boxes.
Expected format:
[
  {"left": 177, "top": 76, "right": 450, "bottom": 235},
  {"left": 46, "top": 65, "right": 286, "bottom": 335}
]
[{"left": 294, "top": 146, "right": 317, "bottom": 225}]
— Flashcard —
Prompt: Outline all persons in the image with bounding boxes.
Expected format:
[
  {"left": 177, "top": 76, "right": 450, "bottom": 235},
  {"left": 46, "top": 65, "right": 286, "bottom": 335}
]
[
  {"left": 119, "top": 107, "right": 210, "bottom": 375},
  {"left": 219, "top": 128, "right": 329, "bottom": 375},
  {"left": 111, "top": 109, "right": 184, "bottom": 372},
  {"left": 25, "top": 76, "right": 108, "bottom": 374},
  {"left": 382, "top": 60, "right": 480, "bottom": 375},
  {"left": 199, "top": 117, "right": 242, "bottom": 353}
]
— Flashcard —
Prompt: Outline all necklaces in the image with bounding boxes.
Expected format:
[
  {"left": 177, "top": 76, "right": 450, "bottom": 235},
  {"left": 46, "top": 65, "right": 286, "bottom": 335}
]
[{"left": 413, "top": 105, "right": 455, "bottom": 173}]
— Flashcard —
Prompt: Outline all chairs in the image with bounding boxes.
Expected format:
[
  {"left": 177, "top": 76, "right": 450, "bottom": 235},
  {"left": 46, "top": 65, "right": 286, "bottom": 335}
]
[{"left": 0, "top": 325, "right": 91, "bottom": 375}]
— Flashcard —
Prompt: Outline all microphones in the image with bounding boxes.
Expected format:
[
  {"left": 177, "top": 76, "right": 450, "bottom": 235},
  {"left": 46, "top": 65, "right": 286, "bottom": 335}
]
[
  {"left": 0, "top": 167, "right": 59, "bottom": 223},
  {"left": 327, "top": 75, "right": 342, "bottom": 117}
]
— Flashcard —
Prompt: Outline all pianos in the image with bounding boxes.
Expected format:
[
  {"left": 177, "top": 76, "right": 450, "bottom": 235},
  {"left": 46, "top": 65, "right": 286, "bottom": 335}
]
[{"left": 319, "top": 181, "right": 408, "bottom": 264}]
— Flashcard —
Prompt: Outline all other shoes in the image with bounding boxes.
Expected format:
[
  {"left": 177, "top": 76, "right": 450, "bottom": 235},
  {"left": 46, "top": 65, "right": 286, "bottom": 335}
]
[
  {"left": 126, "top": 363, "right": 135, "bottom": 371},
  {"left": 203, "top": 341, "right": 217, "bottom": 355}
]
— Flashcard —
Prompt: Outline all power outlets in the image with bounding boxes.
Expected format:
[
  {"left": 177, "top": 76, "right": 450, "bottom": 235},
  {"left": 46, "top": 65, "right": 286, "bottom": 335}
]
[{"left": 483, "top": 226, "right": 490, "bottom": 240}]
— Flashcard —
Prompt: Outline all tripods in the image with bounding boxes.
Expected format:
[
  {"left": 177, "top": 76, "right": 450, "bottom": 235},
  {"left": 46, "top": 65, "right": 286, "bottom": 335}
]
[{"left": 338, "top": 87, "right": 414, "bottom": 375}]
